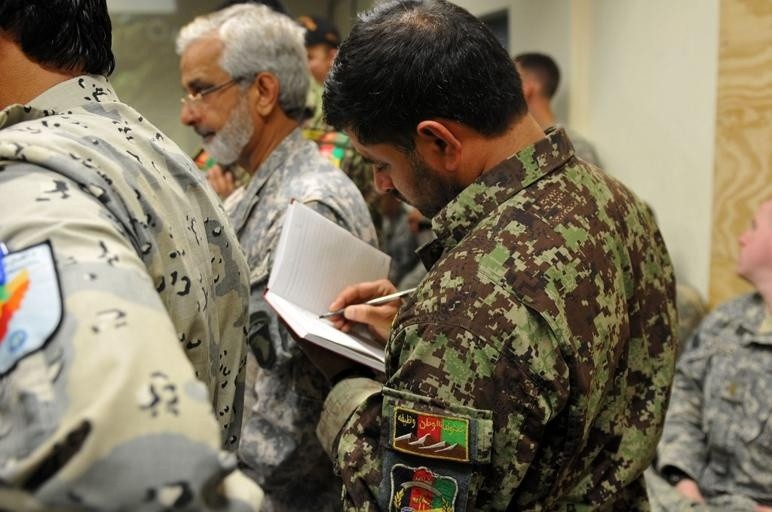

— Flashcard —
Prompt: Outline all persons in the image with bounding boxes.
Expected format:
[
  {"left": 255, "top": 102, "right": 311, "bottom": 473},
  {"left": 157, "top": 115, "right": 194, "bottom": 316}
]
[
  {"left": 651, "top": 198, "right": 772, "bottom": 512},
  {"left": 195, "top": 84, "right": 401, "bottom": 253},
  {"left": 298, "top": 15, "right": 341, "bottom": 85},
  {"left": 0, "top": 0, "right": 252, "bottom": 512},
  {"left": 302, "top": 0, "right": 681, "bottom": 512},
  {"left": 513, "top": 53, "right": 597, "bottom": 166},
  {"left": 173, "top": 3, "right": 391, "bottom": 512}
]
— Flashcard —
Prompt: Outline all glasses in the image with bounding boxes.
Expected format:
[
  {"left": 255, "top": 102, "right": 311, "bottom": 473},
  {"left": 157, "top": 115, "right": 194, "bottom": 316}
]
[{"left": 180, "top": 79, "right": 233, "bottom": 116}]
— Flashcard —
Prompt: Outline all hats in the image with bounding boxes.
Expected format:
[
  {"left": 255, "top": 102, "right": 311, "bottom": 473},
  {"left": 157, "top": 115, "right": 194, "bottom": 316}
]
[{"left": 296, "top": 13, "right": 341, "bottom": 47}]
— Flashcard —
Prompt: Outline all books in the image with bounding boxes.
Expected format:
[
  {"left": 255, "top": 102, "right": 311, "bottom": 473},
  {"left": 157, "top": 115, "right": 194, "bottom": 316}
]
[{"left": 263, "top": 197, "right": 392, "bottom": 374}]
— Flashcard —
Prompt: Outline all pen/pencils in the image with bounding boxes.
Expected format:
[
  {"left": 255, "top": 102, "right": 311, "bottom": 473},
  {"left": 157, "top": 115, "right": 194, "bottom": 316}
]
[{"left": 319, "top": 287, "right": 418, "bottom": 318}]
